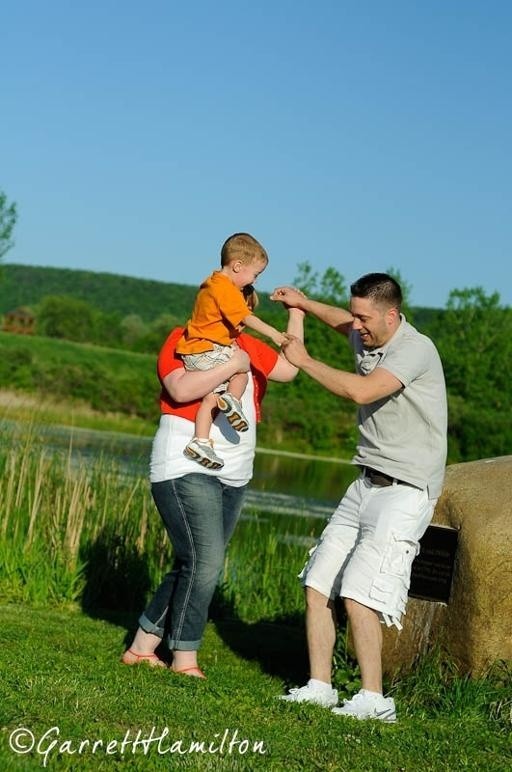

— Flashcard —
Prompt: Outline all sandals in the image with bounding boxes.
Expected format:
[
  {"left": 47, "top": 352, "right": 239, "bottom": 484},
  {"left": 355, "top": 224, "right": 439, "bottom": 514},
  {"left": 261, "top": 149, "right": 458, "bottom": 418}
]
[
  {"left": 121, "top": 649, "right": 169, "bottom": 670},
  {"left": 171, "top": 663, "right": 209, "bottom": 680}
]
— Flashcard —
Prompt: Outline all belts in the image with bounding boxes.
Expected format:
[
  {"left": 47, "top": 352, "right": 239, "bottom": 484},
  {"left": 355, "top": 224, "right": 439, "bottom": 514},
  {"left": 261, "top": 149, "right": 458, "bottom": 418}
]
[{"left": 359, "top": 465, "right": 424, "bottom": 492}]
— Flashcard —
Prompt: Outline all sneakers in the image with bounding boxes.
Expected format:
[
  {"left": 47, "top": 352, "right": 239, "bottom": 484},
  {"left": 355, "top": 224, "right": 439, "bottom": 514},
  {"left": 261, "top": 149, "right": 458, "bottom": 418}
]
[
  {"left": 184, "top": 436, "right": 225, "bottom": 470},
  {"left": 276, "top": 677, "right": 339, "bottom": 709},
  {"left": 329, "top": 689, "right": 397, "bottom": 724},
  {"left": 216, "top": 391, "right": 250, "bottom": 433}
]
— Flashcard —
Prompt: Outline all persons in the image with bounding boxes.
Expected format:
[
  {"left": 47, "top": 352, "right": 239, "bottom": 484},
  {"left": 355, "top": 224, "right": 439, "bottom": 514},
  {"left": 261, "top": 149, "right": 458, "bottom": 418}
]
[
  {"left": 174, "top": 233, "right": 293, "bottom": 468},
  {"left": 270, "top": 271, "right": 449, "bottom": 723},
  {"left": 122, "top": 272, "right": 310, "bottom": 680}
]
